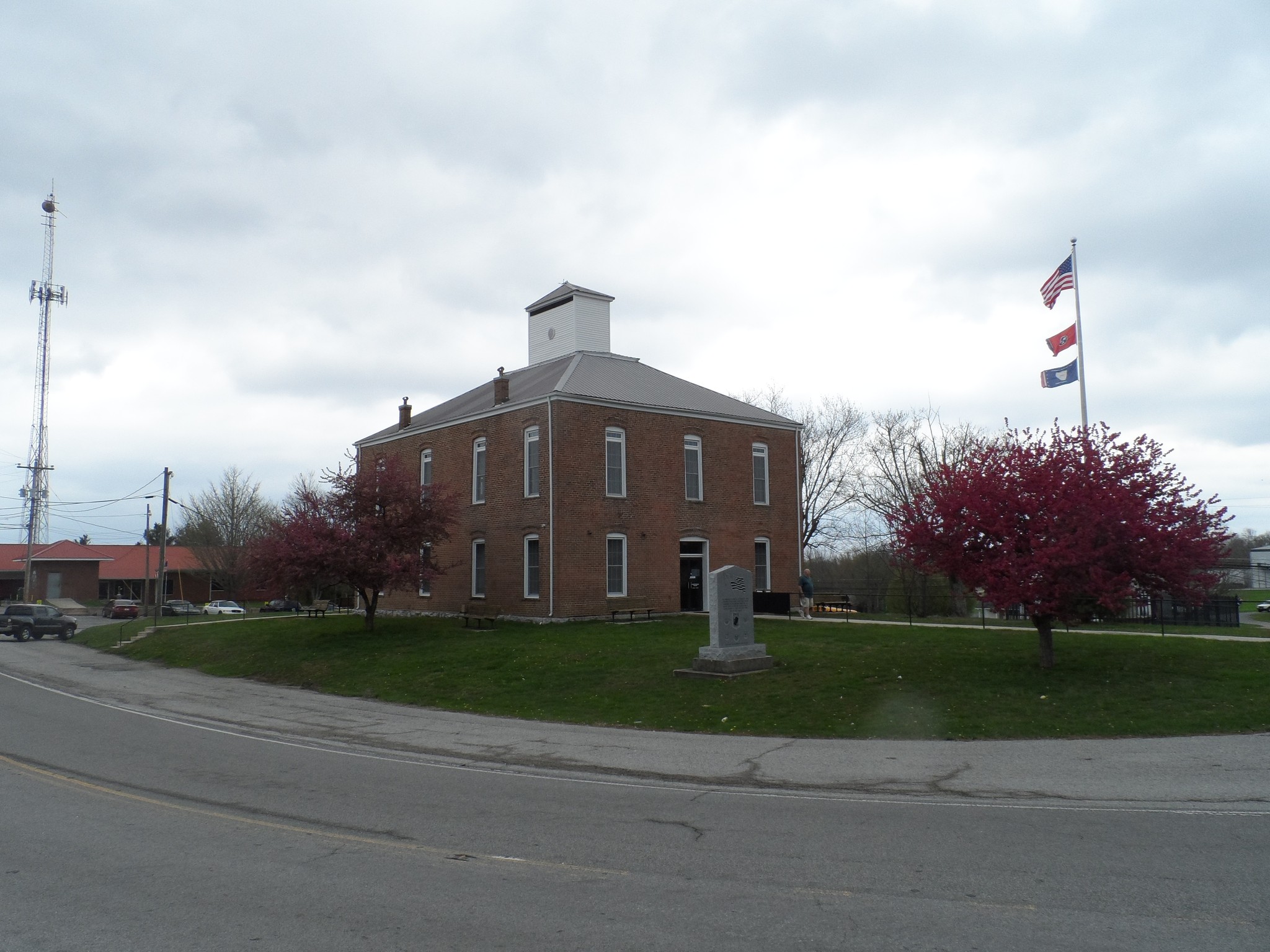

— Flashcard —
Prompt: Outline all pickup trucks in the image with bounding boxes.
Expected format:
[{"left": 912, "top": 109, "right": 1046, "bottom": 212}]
[{"left": 0, "top": 604, "right": 77, "bottom": 642}]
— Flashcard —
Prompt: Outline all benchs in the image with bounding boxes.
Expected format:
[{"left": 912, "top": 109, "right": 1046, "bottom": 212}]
[
  {"left": 813, "top": 595, "right": 852, "bottom": 612},
  {"left": 299, "top": 600, "right": 331, "bottom": 618},
  {"left": 605, "top": 596, "right": 654, "bottom": 622},
  {"left": 455, "top": 603, "right": 502, "bottom": 631}
]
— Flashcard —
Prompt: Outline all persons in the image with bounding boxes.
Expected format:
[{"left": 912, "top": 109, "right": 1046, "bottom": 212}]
[{"left": 797, "top": 569, "right": 813, "bottom": 620}]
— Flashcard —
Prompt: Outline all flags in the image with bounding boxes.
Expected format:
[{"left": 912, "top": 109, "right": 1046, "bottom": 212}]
[
  {"left": 1040, "top": 253, "right": 1075, "bottom": 310},
  {"left": 1046, "top": 322, "right": 1077, "bottom": 357},
  {"left": 1041, "top": 358, "right": 1078, "bottom": 388}
]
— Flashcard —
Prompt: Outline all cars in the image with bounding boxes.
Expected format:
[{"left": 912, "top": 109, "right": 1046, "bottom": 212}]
[
  {"left": 260, "top": 600, "right": 304, "bottom": 612},
  {"left": 1257, "top": 600, "right": 1270, "bottom": 613},
  {"left": 102, "top": 600, "right": 139, "bottom": 618},
  {"left": 202, "top": 600, "right": 246, "bottom": 614},
  {"left": 162, "top": 600, "right": 201, "bottom": 617}
]
